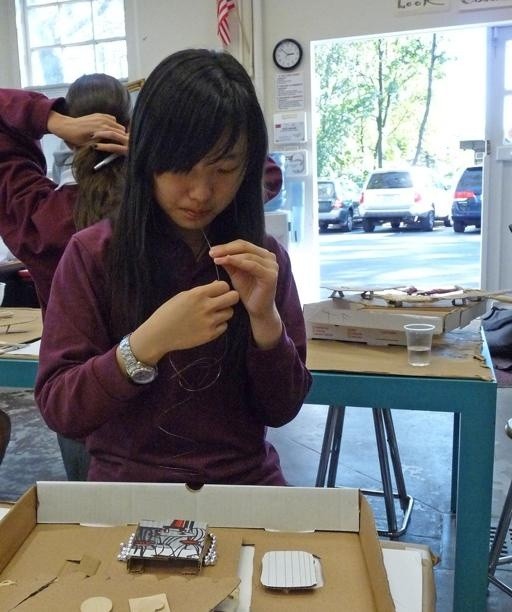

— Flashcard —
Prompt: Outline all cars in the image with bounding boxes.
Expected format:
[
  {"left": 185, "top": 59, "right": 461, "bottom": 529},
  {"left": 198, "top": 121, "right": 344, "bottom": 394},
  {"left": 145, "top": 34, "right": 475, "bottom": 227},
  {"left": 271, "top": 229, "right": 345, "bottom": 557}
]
[
  {"left": 356, "top": 167, "right": 453, "bottom": 231},
  {"left": 318, "top": 179, "right": 358, "bottom": 231},
  {"left": 452, "top": 164, "right": 482, "bottom": 232}
]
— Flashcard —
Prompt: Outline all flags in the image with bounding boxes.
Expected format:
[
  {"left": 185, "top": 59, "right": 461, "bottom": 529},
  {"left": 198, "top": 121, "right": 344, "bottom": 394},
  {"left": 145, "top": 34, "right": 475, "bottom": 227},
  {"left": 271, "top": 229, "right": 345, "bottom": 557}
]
[{"left": 215, "top": 0, "right": 236, "bottom": 51}]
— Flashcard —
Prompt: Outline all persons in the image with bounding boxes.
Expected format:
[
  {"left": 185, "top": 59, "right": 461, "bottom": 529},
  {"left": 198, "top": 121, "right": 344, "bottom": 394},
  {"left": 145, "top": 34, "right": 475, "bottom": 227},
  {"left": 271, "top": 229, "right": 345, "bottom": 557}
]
[
  {"left": 34, "top": 49, "right": 313, "bottom": 488},
  {"left": 2, "top": 75, "right": 138, "bottom": 482}
]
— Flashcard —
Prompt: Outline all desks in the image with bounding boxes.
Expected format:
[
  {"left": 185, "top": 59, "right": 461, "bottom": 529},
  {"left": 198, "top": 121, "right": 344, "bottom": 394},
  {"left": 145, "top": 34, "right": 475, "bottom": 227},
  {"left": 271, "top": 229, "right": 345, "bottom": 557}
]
[
  {"left": 0, "top": 499, "right": 436, "bottom": 612},
  {"left": 0, "top": 298, "right": 497, "bottom": 612}
]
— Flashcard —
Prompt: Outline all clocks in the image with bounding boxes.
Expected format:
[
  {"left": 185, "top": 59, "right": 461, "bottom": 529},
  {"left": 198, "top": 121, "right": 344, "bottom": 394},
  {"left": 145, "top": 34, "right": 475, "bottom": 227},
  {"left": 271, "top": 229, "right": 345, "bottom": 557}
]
[{"left": 273, "top": 39, "right": 302, "bottom": 70}]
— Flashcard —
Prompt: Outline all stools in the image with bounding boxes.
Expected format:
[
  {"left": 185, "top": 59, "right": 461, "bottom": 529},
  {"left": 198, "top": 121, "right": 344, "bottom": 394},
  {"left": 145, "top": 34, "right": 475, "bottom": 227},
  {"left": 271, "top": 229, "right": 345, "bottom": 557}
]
[
  {"left": 315, "top": 403, "right": 413, "bottom": 537},
  {"left": 489, "top": 419, "right": 511, "bottom": 594}
]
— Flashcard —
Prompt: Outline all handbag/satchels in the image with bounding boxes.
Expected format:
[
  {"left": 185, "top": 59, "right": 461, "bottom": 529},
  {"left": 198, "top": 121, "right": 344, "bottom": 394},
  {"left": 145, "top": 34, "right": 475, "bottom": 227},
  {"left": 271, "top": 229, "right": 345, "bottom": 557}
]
[{"left": 481, "top": 307, "right": 512, "bottom": 372}]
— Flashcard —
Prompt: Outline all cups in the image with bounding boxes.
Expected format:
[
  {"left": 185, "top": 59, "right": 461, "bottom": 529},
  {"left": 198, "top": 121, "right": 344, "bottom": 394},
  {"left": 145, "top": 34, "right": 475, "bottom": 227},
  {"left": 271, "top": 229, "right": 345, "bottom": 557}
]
[{"left": 404, "top": 323, "right": 435, "bottom": 366}]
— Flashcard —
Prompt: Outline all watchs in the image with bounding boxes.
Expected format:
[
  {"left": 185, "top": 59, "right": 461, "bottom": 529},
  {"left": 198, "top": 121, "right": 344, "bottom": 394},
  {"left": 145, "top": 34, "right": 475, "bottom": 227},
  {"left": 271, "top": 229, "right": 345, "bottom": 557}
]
[{"left": 120, "top": 336, "right": 158, "bottom": 387}]
